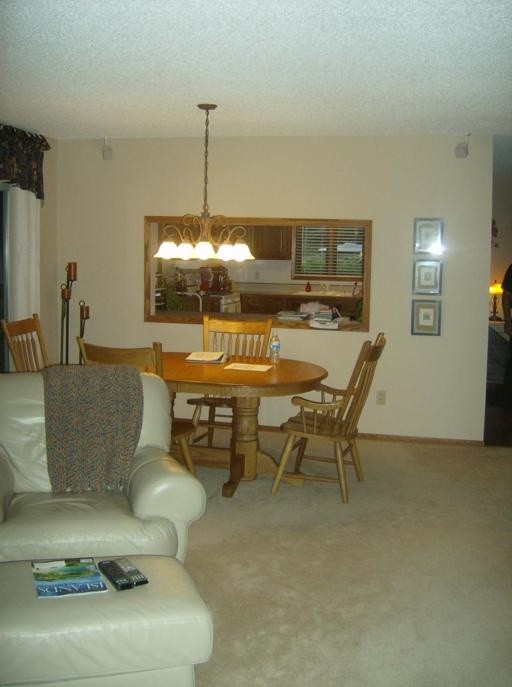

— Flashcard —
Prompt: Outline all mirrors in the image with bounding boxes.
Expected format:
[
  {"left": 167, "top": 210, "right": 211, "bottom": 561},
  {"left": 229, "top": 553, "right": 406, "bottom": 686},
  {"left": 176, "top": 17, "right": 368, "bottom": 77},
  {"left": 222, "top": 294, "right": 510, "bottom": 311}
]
[{"left": 140, "top": 214, "right": 373, "bottom": 332}]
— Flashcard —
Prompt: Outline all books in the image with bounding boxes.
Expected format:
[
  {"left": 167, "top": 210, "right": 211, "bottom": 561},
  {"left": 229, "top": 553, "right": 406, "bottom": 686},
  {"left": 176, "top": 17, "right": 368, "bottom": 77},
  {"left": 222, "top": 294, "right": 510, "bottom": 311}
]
[{"left": 185, "top": 351, "right": 226, "bottom": 364}]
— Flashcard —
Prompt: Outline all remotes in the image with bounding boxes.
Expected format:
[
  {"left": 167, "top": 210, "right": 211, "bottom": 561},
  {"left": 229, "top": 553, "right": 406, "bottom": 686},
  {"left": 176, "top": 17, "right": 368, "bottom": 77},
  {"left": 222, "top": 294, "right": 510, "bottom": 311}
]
[
  {"left": 116, "top": 556, "right": 148, "bottom": 586},
  {"left": 97, "top": 560, "right": 135, "bottom": 591}
]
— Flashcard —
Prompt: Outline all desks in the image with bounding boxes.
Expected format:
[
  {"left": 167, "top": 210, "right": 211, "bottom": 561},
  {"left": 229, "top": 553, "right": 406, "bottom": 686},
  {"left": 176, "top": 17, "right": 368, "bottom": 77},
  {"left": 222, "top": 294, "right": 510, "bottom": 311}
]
[{"left": 144, "top": 348, "right": 327, "bottom": 496}]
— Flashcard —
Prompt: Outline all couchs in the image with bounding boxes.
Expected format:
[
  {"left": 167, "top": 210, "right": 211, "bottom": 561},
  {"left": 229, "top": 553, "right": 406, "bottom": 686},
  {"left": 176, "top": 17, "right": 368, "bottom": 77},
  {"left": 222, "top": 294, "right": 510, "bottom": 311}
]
[
  {"left": 0, "top": 365, "right": 208, "bottom": 565},
  {"left": 0, "top": 555, "right": 217, "bottom": 686}
]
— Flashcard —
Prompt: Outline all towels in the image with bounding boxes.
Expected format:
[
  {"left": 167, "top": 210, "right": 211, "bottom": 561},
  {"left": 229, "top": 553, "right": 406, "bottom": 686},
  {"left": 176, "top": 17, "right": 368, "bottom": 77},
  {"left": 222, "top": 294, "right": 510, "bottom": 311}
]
[{"left": 42, "top": 364, "right": 145, "bottom": 493}]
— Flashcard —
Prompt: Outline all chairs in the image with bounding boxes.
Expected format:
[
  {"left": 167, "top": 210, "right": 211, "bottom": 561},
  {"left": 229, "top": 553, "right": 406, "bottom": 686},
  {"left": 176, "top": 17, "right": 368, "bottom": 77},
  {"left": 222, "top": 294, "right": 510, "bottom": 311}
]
[
  {"left": 182, "top": 312, "right": 275, "bottom": 445},
  {"left": 0, "top": 314, "right": 46, "bottom": 369},
  {"left": 274, "top": 331, "right": 393, "bottom": 500},
  {"left": 78, "top": 333, "right": 199, "bottom": 473}
]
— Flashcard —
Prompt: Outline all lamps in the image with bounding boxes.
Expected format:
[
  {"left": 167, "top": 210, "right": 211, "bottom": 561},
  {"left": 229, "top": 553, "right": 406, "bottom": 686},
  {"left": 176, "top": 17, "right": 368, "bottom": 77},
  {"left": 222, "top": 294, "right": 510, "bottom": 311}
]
[
  {"left": 489, "top": 278, "right": 503, "bottom": 320},
  {"left": 156, "top": 97, "right": 260, "bottom": 263}
]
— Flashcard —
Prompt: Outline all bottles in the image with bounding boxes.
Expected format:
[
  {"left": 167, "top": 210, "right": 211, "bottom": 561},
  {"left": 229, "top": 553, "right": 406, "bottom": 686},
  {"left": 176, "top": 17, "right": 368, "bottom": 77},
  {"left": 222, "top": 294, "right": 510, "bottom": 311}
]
[
  {"left": 355, "top": 299, "right": 363, "bottom": 321},
  {"left": 269, "top": 332, "right": 281, "bottom": 363}
]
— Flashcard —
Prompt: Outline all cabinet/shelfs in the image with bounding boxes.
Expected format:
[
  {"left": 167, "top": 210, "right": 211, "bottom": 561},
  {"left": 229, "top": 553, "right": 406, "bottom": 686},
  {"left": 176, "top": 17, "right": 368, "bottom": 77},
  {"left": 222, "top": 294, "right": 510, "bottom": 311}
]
[
  {"left": 251, "top": 223, "right": 291, "bottom": 259},
  {"left": 242, "top": 292, "right": 353, "bottom": 315}
]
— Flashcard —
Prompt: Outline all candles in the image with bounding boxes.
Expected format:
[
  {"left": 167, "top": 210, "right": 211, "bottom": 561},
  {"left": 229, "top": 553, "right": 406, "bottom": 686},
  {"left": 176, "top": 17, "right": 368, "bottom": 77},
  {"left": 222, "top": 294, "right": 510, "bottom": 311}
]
[
  {"left": 61, "top": 287, "right": 70, "bottom": 298},
  {"left": 66, "top": 262, "right": 77, "bottom": 280},
  {"left": 80, "top": 305, "right": 88, "bottom": 316}
]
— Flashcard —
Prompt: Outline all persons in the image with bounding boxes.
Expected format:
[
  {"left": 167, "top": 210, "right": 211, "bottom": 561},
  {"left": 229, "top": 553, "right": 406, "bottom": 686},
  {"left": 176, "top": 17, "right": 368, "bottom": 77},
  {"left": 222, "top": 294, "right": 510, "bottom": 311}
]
[
  {"left": 31, "top": 557, "right": 109, "bottom": 598},
  {"left": 502, "top": 263, "right": 512, "bottom": 337}
]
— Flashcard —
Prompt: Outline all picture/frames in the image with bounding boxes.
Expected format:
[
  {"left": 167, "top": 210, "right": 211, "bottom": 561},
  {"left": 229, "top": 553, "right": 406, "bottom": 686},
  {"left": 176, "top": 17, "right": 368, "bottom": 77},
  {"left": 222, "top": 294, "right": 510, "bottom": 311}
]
[
  {"left": 412, "top": 217, "right": 446, "bottom": 257},
  {"left": 410, "top": 296, "right": 442, "bottom": 336},
  {"left": 413, "top": 260, "right": 443, "bottom": 294}
]
